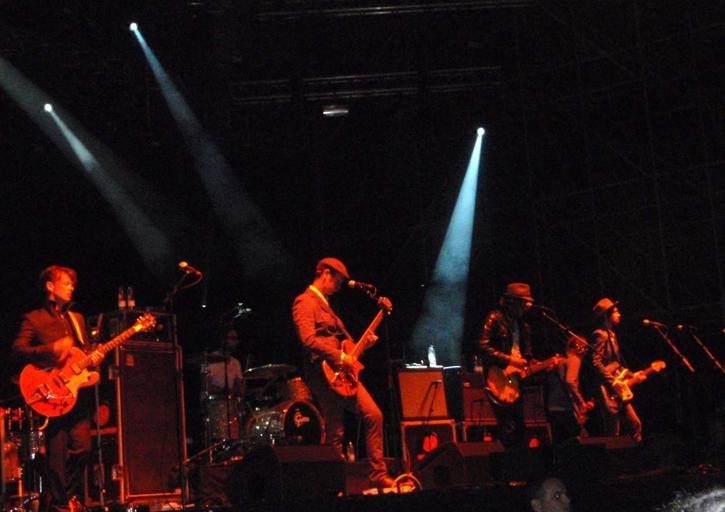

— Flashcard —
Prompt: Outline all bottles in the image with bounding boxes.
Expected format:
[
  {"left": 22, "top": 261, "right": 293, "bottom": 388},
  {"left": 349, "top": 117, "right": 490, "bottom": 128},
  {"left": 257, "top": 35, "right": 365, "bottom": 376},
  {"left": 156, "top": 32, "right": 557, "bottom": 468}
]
[{"left": 427, "top": 343, "right": 436, "bottom": 368}]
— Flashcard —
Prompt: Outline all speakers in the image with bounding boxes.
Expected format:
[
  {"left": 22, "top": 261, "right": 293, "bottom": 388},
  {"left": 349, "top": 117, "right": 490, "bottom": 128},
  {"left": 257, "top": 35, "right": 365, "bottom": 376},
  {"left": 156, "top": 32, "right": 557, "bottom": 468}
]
[
  {"left": 223, "top": 445, "right": 346, "bottom": 507},
  {"left": 527, "top": 437, "right": 634, "bottom": 486},
  {"left": 88, "top": 340, "right": 189, "bottom": 506},
  {"left": 388, "top": 418, "right": 458, "bottom": 473},
  {"left": 413, "top": 442, "right": 508, "bottom": 491},
  {"left": 455, "top": 422, "right": 499, "bottom": 442}
]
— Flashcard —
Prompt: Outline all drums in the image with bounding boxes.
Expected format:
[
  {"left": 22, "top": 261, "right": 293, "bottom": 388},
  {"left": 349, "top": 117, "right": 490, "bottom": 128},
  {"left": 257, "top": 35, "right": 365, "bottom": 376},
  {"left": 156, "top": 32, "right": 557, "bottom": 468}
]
[
  {"left": 200, "top": 397, "right": 244, "bottom": 466},
  {"left": 246, "top": 400, "right": 327, "bottom": 447},
  {"left": 288, "top": 377, "right": 313, "bottom": 402}
]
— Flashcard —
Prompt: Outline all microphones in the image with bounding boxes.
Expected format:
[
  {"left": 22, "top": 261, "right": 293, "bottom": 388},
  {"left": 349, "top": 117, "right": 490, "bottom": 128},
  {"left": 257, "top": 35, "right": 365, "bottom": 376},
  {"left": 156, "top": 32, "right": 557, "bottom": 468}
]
[
  {"left": 677, "top": 324, "right": 697, "bottom": 329},
  {"left": 525, "top": 302, "right": 551, "bottom": 312},
  {"left": 348, "top": 280, "right": 373, "bottom": 289},
  {"left": 178, "top": 261, "right": 201, "bottom": 277},
  {"left": 643, "top": 318, "right": 665, "bottom": 328}
]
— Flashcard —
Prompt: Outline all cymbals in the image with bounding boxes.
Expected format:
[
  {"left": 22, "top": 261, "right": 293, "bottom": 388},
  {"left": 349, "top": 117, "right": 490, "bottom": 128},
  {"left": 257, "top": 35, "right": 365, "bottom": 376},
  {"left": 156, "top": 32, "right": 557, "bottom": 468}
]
[{"left": 242, "top": 364, "right": 295, "bottom": 378}]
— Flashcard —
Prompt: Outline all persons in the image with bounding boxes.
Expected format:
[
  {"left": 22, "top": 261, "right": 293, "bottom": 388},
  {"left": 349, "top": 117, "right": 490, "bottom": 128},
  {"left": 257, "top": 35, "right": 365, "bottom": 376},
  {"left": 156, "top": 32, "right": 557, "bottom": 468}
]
[
  {"left": 522, "top": 472, "right": 579, "bottom": 512},
  {"left": 197, "top": 320, "right": 246, "bottom": 446},
  {"left": 586, "top": 296, "right": 652, "bottom": 443},
  {"left": 12, "top": 262, "right": 109, "bottom": 511},
  {"left": 472, "top": 281, "right": 566, "bottom": 445},
  {"left": 556, "top": 328, "right": 590, "bottom": 438},
  {"left": 652, "top": 481, "right": 725, "bottom": 512},
  {"left": 291, "top": 254, "right": 408, "bottom": 491}
]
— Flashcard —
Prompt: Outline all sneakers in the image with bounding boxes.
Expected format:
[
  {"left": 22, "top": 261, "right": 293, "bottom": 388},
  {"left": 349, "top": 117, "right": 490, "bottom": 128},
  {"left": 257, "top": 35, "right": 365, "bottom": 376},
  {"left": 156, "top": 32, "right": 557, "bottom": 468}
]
[{"left": 367, "top": 474, "right": 394, "bottom": 488}]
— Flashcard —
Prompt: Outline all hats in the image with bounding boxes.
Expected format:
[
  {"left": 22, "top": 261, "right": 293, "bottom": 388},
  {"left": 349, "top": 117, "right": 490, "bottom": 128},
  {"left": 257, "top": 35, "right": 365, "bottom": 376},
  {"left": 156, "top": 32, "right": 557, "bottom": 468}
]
[
  {"left": 315, "top": 257, "right": 351, "bottom": 280},
  {"left": 592, "top": 297, "right": 619, "bottom": 319},
  {"left": 502, "top": 283, "right": 534, "bottom": 301}
]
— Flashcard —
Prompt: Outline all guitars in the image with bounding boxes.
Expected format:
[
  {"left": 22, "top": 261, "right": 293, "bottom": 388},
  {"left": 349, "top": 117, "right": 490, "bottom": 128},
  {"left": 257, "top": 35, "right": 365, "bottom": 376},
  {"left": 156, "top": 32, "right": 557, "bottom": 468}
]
[
  {"left": 600, "top": 361, "right": 666, "bottom": 415},
  {"left": 19, "top": 314, "right": 156, "bottom": 418},
  {"left": 485, "top": 346, "right": 586, "bottom": 403},
  {"left": 322, "top": 296, "right": 392, "bottom": 398}
]
